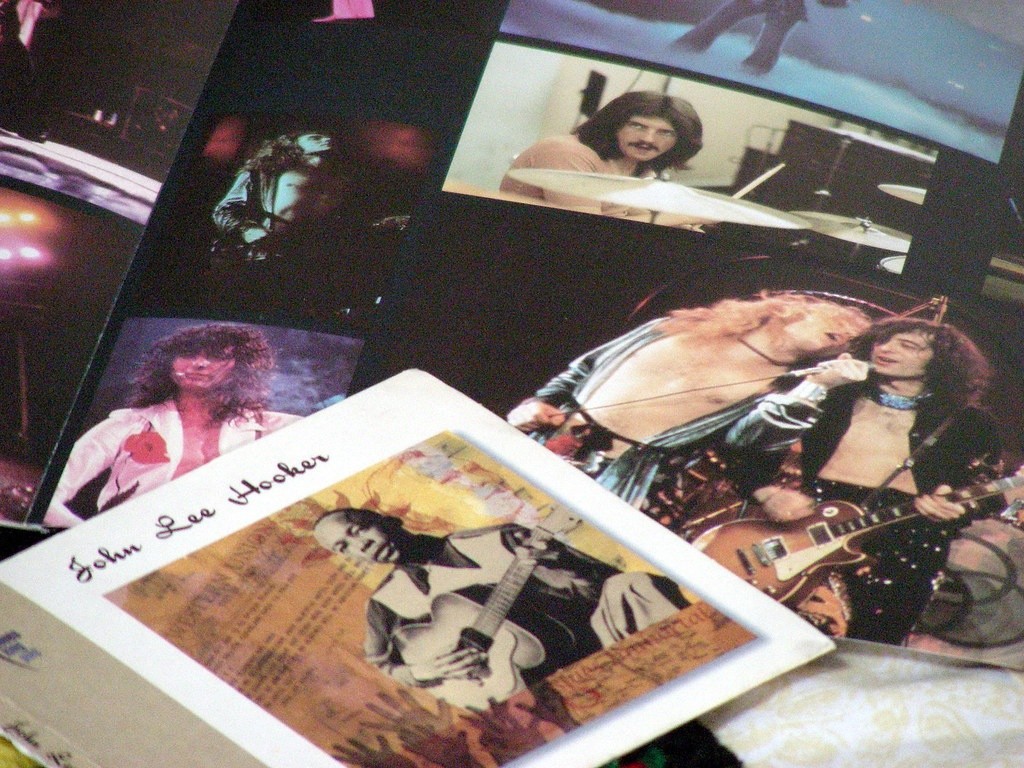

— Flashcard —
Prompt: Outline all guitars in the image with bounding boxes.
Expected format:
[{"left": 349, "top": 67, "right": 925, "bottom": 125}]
[
  {"left": 391, "top": 503, "right": 583, "bottom": 713},
  {"left": 675, "top": 454, "right": 1024, "bottom": 607}
]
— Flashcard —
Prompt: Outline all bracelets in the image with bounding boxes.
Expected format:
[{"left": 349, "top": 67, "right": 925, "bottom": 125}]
[
  {"left": 758, "top": 489, "right": 781, "bottom": 506},
  {"left": 790, "top": 381, "right": 826, "bottom": 402}
]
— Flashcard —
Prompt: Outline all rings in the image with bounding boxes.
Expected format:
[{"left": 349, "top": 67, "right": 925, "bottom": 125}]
[{"left": 780, "top": 512, "right": 792, "bottom": 522}]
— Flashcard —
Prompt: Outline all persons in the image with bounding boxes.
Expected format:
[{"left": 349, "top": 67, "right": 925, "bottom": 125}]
[
  {"left": 664, "top": 0, "right": 808, "bottom": 74},
  {"left": 505, "top": 293, "right": 1008, "bottom": 645},
  {"left": 212, "top": 124, "right": 368, "bottom": 277},
  {"left": 41, "top": 322, "right": 308, "bottom": 530},
  {"left": 312, "top": 507, "right": 692, "bottom": 715},
  {"left": 499, "top": 92, "right": 704, "bottom": 227}
]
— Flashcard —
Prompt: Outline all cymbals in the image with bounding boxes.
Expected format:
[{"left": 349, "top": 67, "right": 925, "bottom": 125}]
[
  {"left": 787, "top": 206, "right": 913, "bottom": 257},
  {"left": 504, "top": 163, "right": 810, "bottom": 233},
  {"left": 872, "top": 180, "right": 924, "bottom": 207}
]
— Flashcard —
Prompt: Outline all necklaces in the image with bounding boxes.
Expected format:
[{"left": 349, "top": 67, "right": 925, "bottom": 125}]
[
  {"left": 861, "top": 384, "right": 935, "bottom": 410},
  {"left": 737, "top": 335, "right": 794, "bottom": 368}
]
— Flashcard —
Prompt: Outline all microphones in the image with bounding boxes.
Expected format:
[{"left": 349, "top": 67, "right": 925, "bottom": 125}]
[{"left": 788, "top": 358, "right": 877, "bottom": 377}]
